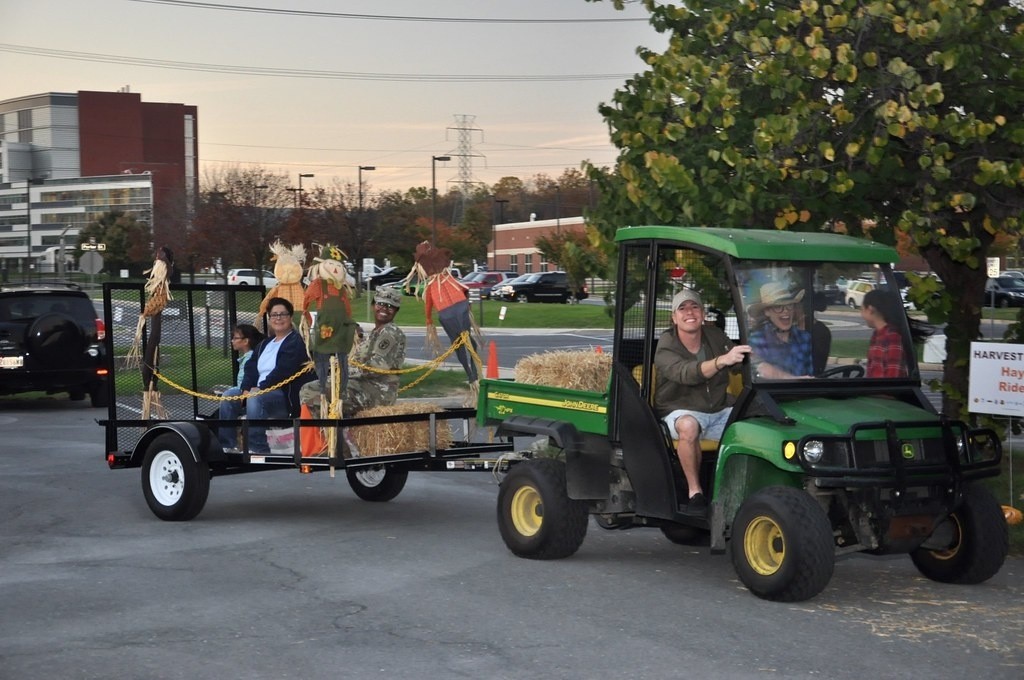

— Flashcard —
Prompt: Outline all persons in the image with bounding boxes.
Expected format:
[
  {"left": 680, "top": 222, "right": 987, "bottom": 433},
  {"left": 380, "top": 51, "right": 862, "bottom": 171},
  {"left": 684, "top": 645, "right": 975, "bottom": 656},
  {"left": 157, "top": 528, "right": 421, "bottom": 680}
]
[
  {"left": 299, "top": 286, "right": 405, "bottom": 457},
  {"left": 703, "top": 303, "right": 726, "bottom": 332},
  {"left": 861, "top": 289, "right": 933, "bottom": 378},
  {"left": 652, "top": 290, "right": 817, "bottom": 513},
  {"left": 195, "top": 324, "right": 263, "bottom": 422},
  {"left": 746, "top": 281, "right": 818, "bottom": 379},
  {"left": 790, "top": 285, "right": 831, "bottom": 377},
  {"left": 218, "top": 297, "right": 308, "bottom": 454}
]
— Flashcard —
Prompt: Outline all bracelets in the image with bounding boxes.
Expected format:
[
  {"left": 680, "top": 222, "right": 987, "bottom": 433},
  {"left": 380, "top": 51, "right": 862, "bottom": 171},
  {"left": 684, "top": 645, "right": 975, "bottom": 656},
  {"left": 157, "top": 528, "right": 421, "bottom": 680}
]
[{"left": 715, "top": 356, "right": 721, "bottom": 371}]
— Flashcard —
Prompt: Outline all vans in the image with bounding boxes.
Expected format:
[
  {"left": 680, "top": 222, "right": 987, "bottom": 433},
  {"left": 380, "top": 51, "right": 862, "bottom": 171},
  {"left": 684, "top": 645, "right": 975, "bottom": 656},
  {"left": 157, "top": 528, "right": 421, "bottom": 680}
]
[{"left": 226, "top": 269, "right": 276, "bottom": 289}]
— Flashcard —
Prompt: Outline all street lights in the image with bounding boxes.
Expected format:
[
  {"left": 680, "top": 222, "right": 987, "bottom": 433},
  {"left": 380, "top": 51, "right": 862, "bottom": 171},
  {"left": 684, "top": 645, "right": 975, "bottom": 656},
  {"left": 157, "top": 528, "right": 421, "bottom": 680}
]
[
  {"left": 299, "top": 173, "right": 314, "bottom": 227},
  {"left": 58, "top": 225, "right": 72, "bottom": 282},
  {"left": 254, "top": 186, "right": 267, "bottom": 220},
  {"left": 495, "top": 198, "right": 510, "bottom": 223},
  {"left": 433, "top": 157, "right": 451, "bottom": 246},
  {"left": 553, "top": 186, "right": 559, "bottom": 271},
  {"left": 27, "top": 178, "right": 45, "bottom": 287},
  {"left": 359, "top": 166, "right": 375, "bottom": 268},
  {"left": 285, "top": 188, "right": 304, "bottom": 208}
]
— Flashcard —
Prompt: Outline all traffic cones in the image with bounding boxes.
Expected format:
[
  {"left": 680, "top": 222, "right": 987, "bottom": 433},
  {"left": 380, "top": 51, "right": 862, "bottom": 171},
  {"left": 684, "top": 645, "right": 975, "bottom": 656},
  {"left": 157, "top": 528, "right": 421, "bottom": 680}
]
[{"left": 484, "top": 341, "right": 501, "bottom": 377}]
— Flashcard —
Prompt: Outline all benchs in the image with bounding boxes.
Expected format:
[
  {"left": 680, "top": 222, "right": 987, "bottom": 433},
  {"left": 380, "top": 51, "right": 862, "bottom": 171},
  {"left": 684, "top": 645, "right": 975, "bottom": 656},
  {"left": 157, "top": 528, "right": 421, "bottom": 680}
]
[{"left": 632, "top": 361, "right": 745, "bottom": 452}]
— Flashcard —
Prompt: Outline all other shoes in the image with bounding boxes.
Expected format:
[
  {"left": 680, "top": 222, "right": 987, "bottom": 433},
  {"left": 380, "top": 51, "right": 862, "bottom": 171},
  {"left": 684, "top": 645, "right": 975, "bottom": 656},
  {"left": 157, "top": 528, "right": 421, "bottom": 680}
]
[{"left": 686, "top": 492, "right": 706, "bottom": 517}]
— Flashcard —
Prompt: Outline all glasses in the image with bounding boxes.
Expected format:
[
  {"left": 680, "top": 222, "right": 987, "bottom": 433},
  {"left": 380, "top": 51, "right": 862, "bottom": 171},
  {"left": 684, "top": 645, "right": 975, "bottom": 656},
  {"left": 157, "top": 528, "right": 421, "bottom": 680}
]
[
  {"left": 233, "top": 337, "right": 244, "bottom": 341},
  {"left": 374, "top": 301, "right": 392, "bottom": 308},
  {"left": 270, "top": 311, "right": 290, "bottom": 320},
  {"left": 768, "top": 304, "right": 795, "bottom": 313}
]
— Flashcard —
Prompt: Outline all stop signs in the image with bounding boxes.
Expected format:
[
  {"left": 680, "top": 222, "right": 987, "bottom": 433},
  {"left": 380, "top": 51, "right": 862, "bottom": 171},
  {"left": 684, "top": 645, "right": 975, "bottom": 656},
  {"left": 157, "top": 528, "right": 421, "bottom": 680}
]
[{"left": 670, "top": 262, "right": 687, "bottom": 281}]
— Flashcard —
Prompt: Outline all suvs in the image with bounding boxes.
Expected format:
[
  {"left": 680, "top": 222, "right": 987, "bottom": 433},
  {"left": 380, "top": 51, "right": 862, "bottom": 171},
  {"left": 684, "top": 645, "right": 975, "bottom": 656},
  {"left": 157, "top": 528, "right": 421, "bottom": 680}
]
[
  {"left": 459, "top": 272, "right": 520, "bottom": 288},
  {"left": 499, "top": 270, "right": 589, "bottom": 306},
  {"left": 0, "top": 282, "right": 108, "bottom": 409}
]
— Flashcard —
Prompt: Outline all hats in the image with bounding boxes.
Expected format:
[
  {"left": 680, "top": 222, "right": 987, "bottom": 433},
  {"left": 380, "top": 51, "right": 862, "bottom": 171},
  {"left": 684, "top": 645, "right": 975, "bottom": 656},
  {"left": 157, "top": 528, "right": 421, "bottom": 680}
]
[
  {"left": 748, "top": 281, "right": 805, "bottom": 321},
  {"left": 672, "top": 289, "right": 701, "bottom": 311},
  {"left": 374, "top": 286, "right": 402, "bottom": 308}
]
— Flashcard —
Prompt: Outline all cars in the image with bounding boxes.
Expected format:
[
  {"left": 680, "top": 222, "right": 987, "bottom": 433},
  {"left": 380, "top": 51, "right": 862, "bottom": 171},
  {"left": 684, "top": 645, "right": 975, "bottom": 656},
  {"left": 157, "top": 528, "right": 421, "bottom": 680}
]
[
  {"left": 826, "top": 270, "right": 1024, "bottom": 310},
  {"left": 363, "top": 265, "right": 410, "bottom": 289},
  {"left": 482, "top": 274, "right": 534, "bottom": 299}
]
